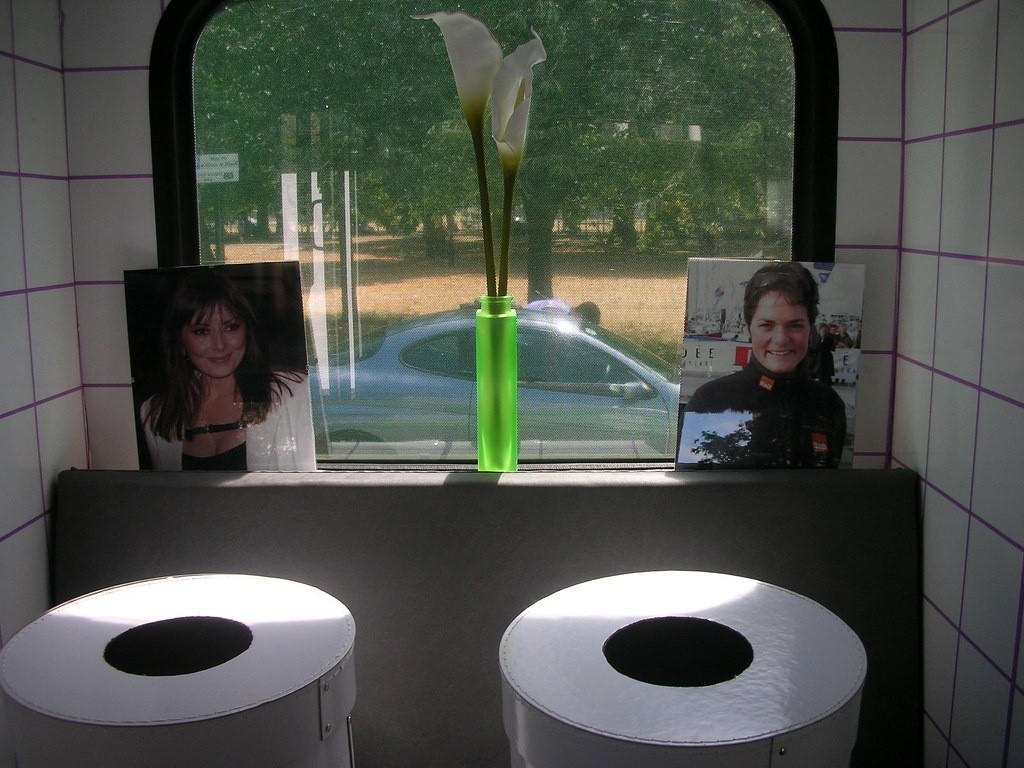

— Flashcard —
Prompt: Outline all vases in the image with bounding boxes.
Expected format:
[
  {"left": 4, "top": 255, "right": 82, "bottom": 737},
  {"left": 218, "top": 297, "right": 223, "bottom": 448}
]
[{"left": 473, "top": 298, "right": 520, "bottom": 472}]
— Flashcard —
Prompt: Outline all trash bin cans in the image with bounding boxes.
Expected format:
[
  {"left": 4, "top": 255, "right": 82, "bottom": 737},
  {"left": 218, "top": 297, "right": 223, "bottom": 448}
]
[
  {"left": 498, "top": 569, "right": 868, "bottom": 768},
  {"left": 0, "top": 572, "right": 356, "bottom": 768}
]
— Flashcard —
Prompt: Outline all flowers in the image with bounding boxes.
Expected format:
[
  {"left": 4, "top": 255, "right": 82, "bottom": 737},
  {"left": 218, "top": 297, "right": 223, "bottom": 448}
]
[{"left": 407, "top": 11, "right": 548, "bottom": 295}]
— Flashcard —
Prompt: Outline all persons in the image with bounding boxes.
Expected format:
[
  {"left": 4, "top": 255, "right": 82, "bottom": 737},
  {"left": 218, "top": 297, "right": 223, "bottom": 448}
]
[
  {"left": 529, "top": 299, "right": 600, "bottom": 323},
  {"left": 140, "top": 270, "right": 317, "bottom": 471},
  {"left": 676, "top": 262, "right": 862, "bottom": 468}
]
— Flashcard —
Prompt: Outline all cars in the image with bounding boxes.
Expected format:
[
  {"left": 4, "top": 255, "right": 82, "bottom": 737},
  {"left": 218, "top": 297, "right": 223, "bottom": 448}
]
[{"left": 309, "top": 297, "right": 674, "bottom": 461}]
